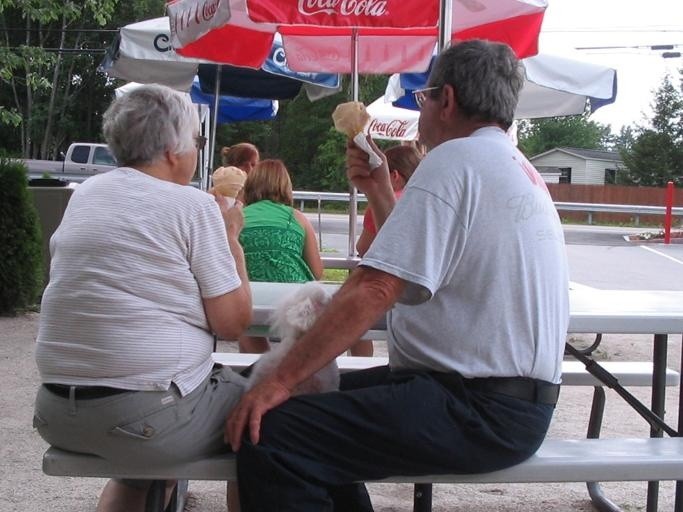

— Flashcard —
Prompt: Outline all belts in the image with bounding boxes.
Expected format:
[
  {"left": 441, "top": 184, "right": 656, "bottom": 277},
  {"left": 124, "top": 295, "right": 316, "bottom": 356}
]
[
  {"left": 464, "top": 375, "right": 559, "bottom": 406},
  {"left": 42, "top": 382, "right": 132, "bottom": 401}
]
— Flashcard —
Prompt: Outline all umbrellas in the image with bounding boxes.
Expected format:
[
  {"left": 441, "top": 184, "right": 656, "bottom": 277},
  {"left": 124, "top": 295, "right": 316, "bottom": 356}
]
[
  {"left": 387, "top": 40, "right": 620, "bottom": 131},
  {"left": 165, "top": 0, "right": 549, "bottom": 257},
  {"left": 101, "top": 59, "right": 281, "bottom": 191},
  {"left": 113, "top": 11, "right": 346, "bottom": 194},
  {"left": 356, "top": 82, "right": 518, "bottom": 151}
]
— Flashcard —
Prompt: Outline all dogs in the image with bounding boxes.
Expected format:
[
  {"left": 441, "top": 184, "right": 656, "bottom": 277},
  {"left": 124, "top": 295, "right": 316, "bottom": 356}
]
[{"left": 243, "top": 280, "right": 340, "bottom": 398}]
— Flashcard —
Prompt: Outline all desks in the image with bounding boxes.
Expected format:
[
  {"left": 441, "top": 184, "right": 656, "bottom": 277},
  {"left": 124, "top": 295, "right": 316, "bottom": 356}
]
[{"left": 202, "top": 277, "right": 683, "bottom": 506}]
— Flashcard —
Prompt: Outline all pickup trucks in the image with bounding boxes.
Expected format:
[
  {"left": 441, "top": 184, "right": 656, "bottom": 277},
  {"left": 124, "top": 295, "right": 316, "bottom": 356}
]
[{"left": 1, "top": 143, "right": 119, "bottom": 189}]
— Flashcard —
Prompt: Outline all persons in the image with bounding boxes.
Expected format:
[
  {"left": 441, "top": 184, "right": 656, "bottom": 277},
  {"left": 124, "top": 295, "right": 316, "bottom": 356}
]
[
  {"left": 31, "top": 80, "right": 255, "bottom": 510},
  {"left": 343, "top": 135, "right": 426, "bottom": 357},
  {"left": 222, "top": 38, "right": 571, "bottom": 511},
  {"left": 238, "top": 158, "right": 324, "bottom": 353},
  {"left": 220, "top": 142, "right": 259, "bottom": 208}
]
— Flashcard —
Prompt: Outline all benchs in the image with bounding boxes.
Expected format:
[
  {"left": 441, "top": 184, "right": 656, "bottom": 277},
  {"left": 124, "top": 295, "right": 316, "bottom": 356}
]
[
  {"left": 45, "top": 434, "right": 683, "bottom": 507},
  {"left": 208, "top": 352, "right": 676, "bottom": 505}
]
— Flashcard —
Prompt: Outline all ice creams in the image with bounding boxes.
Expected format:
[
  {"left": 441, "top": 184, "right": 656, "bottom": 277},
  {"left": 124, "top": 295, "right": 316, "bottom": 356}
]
[
  {"left": 211, "top": 165, "right": 248, "bottom": 198},
  {"left": 331, "top": 100, "right": 370, "bottom": 137}
]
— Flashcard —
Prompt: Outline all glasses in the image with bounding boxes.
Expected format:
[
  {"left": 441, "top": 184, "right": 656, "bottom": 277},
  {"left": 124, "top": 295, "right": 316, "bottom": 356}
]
[
  {"left": 411, "top": 85, "right": 439, "bottom": 110},
  {"left": 192, "top": 135, "right": 207, "bottom": 150}
]
